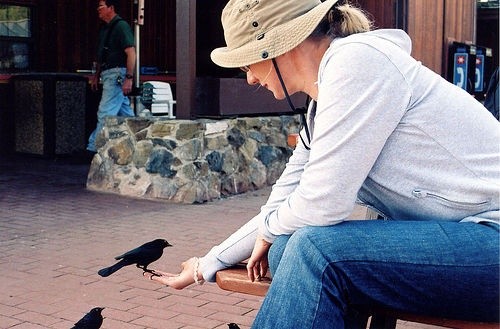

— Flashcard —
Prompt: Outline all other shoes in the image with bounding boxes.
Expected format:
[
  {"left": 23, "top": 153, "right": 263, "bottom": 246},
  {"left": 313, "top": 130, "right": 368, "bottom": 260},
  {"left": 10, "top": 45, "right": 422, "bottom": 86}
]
[{"left": 80, "top": 151, "right": 95, "bottom": 162}]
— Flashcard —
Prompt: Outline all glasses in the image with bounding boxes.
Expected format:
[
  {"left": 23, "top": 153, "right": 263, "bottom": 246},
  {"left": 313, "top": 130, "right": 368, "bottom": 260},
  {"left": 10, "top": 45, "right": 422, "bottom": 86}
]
[{"left": 240, "top": 66, "right": 250, "bottom": 72}]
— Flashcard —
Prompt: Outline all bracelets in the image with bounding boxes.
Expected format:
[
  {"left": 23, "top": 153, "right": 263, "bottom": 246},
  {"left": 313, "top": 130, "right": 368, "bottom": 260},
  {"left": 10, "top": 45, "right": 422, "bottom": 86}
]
[{"left": 193, "top": 257, "right": 205, "bottom": 286}]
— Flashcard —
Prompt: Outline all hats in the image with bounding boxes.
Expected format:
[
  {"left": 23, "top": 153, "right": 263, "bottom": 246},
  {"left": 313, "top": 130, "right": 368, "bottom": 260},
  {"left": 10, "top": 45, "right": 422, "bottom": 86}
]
[{"left": 210, "top": 0, "right": 339, "bottom": 68}]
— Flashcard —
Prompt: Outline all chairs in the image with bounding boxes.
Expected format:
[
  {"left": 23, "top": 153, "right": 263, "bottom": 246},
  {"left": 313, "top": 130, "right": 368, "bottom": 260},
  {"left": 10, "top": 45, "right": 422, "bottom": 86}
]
[{"left": 143, "top": 81, "right": 177, "bottom": 120}]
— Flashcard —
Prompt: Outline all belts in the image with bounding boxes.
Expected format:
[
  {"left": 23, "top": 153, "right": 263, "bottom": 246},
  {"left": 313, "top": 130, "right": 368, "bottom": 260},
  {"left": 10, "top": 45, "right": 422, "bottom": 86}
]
[{"left": 101, "top": 63, "right": 125, "bottom": 70}]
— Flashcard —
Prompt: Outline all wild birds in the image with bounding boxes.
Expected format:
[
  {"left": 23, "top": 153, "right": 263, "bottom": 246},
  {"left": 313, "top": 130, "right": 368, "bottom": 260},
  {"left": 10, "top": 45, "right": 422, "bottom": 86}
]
[
  {"left": 227, "top": 323, "right": 241, "bottom": 329},
  {"left": 69, "top": 307, "right": 106, "bottom": 329},
  {"left": 98, "top": 239, "right": 174, "bottom": 280}
]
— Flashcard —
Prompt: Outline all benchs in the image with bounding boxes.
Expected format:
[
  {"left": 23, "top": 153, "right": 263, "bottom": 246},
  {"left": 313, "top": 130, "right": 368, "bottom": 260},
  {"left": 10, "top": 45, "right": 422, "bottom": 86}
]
[{"left": 216, "top": 265, "right": 500, "bottom": 329}]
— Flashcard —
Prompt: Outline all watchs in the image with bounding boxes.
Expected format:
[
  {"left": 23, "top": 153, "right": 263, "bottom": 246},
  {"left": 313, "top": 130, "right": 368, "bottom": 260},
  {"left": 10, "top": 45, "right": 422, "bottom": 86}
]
[{"left": 126, "top": 74, "right": 133, "bottom": 79}]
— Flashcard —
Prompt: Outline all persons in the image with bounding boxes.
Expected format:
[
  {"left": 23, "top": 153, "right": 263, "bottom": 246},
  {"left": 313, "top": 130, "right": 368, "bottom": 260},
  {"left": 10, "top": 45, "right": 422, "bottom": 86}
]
[
  {"left": 73, "top": 0, "right": 135, "bottom": 164},
  {"left": 142, "top": 0, "right": 500, "bottom": 329}
]
